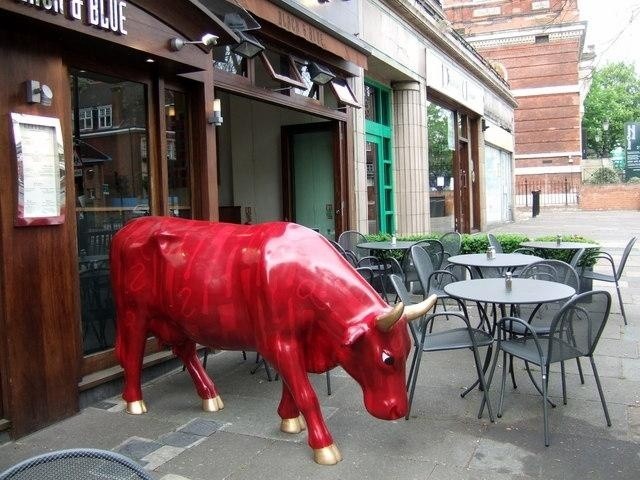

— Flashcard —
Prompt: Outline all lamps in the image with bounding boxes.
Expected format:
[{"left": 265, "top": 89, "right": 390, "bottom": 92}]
[
  {"left": 306, "top": 61, "right": 335, "bottom": 85},
  {"left": 172, "top": 33, "right": 218, "bottom": 53},
  {"left": 231, "top": 33, "right": 265, "bottom": 60},
  {"left": 207, "top": 97, "right": 223, "bottom": 128}
]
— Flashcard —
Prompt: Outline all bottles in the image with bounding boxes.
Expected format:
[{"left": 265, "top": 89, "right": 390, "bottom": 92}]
[
  {"left": 557, "top": 234, "right": 562, "bottom": 244},
  {"left": 505, "top": 271, "right": 512, "bottom": 290},
  {"left": 486, "top": 246, "right": 495, "bottom": 259},
  {"left": 390, "top": 234, "right": 396, "bottom": 244},
  {"left": 88, "top": 233, "right": 113, "bottom": 254}
]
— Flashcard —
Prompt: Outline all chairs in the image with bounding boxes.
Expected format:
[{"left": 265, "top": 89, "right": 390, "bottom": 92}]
[{"left": 1, "top": 449, "right": 155, "bottom": 478}]
